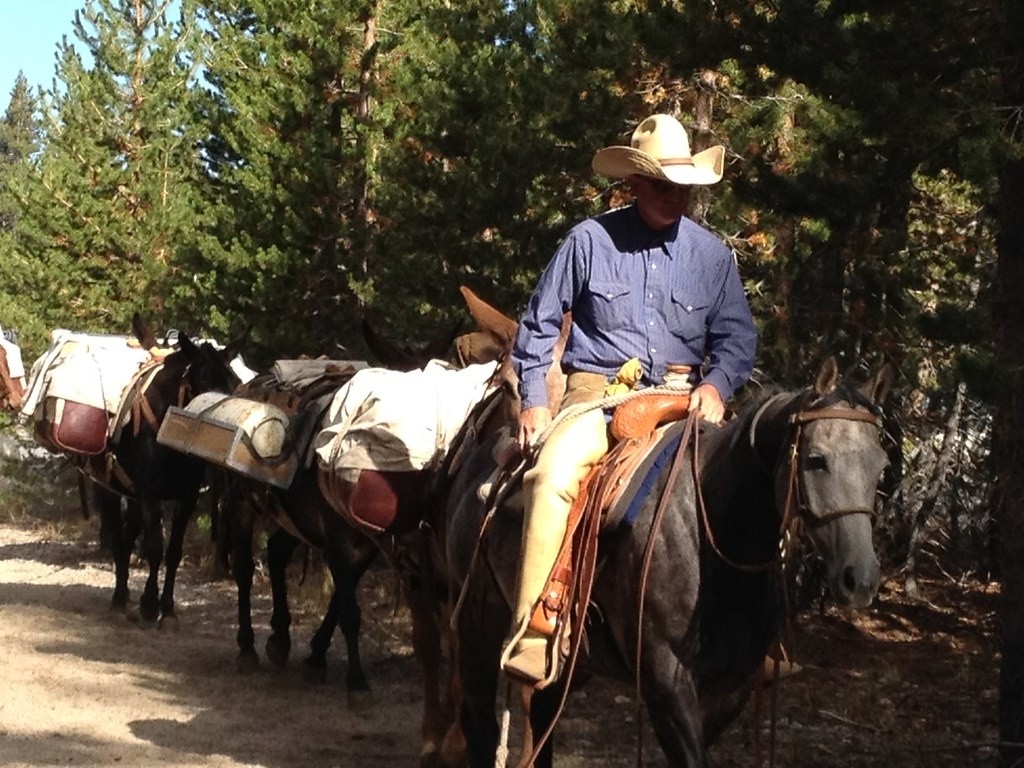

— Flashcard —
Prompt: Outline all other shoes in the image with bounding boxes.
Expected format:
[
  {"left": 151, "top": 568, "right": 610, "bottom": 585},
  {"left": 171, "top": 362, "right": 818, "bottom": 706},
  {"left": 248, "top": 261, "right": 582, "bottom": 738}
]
[{"left": 504, "top": 644, "right": 550, "bottom": 680}]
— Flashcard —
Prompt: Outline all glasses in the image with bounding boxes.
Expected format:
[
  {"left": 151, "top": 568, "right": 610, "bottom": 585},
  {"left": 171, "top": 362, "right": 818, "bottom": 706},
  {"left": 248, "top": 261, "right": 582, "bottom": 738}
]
[{"left": 643, "top": 177, "right": 693, "bottom": 193}]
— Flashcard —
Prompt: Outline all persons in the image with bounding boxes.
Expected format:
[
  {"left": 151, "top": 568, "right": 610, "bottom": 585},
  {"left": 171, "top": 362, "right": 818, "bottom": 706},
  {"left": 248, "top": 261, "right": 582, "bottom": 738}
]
[{"left": 502, "top": 113, "right": 758, "bottom": 682}]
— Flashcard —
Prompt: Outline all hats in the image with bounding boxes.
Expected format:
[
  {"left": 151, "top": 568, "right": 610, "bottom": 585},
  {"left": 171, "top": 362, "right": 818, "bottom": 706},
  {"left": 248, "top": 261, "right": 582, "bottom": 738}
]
[{"left": 592, "top": 114, "right": 725, "bottom": 185}]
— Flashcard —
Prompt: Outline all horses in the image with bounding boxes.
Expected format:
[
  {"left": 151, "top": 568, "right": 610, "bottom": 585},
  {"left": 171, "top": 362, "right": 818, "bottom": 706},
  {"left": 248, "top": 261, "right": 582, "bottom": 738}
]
[{"left": 0, "top": 284, "right": 896, "bottom": 768}]
